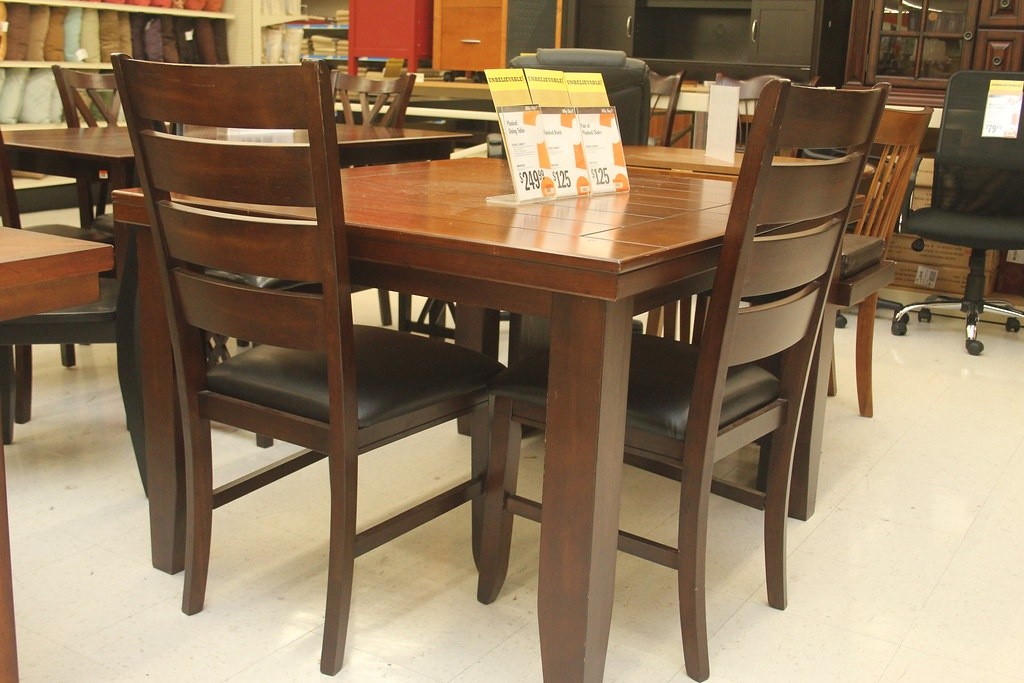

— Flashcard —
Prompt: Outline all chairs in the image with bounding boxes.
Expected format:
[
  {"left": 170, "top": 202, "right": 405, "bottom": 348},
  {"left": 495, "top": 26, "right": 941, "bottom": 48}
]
[
  {"left": 650, "top": 64, "right": 686, "bottom": 145},
  {"left": 470, "top": 73, "right": 887, "bottom": 683},
  {"left": 96, "top": 48, "right": 503, "bottom": 676},
  {"left": 887, "top": 68, "right": 1024, "bottom": 354},
  {"left": 705, "top": 66, "right": 822, "bottom": 152},
  {"left": 0, "top": 115, "right": 119, "bottom": 248},
  {"left": 0, "top": 121, "right": 183, "bottom": 483},
  {"left": 328, "top": 70, "right": 415, "bottom": 126},
  {"left": 510, "top": 46, "right": 656, "bottom": 148},
  {"left": 829, "top": 88, "right": 934, "bottom": 419},
  {"left": 50, "top": 61, "right": 123, "bottom": 240}
]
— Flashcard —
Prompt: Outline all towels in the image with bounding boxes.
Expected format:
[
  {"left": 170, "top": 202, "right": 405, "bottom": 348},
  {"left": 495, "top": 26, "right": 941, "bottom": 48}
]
[{"left": 302, "top": 11, "right": 348, "bottom": 55}]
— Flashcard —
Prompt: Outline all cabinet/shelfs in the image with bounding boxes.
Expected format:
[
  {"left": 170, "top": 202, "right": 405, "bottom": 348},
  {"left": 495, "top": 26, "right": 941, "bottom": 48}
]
[
  {"left": 345, "top": 0, "right": 434, "bottom": 74},
  {"left": 429, "top": 0, "right": 557, "bottom": 73},
  {"left": 1, "top": 1, "right": 242, "bottom": 123}
]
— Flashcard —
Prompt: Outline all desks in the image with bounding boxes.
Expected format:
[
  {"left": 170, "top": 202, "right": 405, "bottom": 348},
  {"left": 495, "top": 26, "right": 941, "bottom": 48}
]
[
  {"left": 107, "top": 154, "right": 746, "bottom": 683},
  {"left": 0, "top": 226, "right": 114, "bottom": 683},
  {"left": 7, "top": 117, "right": 470, "bottom": 425},
  {"left": 576, "top": 0, "right": 852, "bottom": 86}
]
